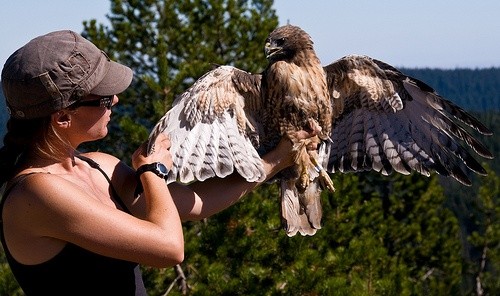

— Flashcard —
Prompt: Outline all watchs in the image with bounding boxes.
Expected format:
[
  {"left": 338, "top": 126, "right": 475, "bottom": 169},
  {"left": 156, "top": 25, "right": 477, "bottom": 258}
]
[{"left": 137, "top": 162, "right": 168, "bottom": 180}]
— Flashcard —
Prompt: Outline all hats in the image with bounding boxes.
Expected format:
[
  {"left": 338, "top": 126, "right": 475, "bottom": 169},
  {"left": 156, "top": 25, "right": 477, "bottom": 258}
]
[{"left": 1, "top": 28, "right": 135, "bottom": 120}]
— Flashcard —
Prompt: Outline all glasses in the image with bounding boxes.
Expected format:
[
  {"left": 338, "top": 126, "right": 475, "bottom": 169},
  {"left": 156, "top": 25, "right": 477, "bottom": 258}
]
[{"left": 72, "top": 94, "right": 114, "bottom": 110}]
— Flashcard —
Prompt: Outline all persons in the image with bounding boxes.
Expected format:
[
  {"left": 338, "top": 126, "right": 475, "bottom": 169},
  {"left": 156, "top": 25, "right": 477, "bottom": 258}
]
[{"left": 0, "top": 30, "right": 322, "bottom": 296}]
